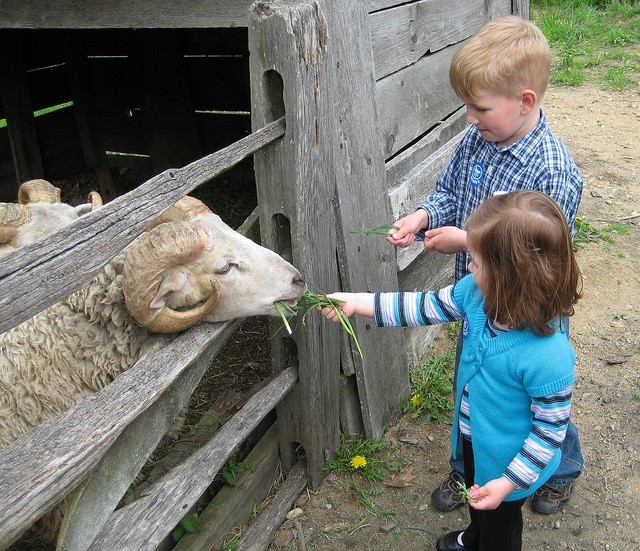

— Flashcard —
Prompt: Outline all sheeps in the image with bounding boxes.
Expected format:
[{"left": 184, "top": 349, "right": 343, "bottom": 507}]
[{"left": 0, "top": 177, "right": 309, "bottom": 551}]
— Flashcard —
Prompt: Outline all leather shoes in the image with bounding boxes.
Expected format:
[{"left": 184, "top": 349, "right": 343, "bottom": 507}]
[{"left": 436, "top": 529, "right": 467, "bottom": 551}]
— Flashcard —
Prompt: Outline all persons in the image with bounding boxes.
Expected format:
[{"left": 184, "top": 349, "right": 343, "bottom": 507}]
[
  {"left": 386, "top": 14, "right": 584, "bottom": 515},
  {"left": 315, "top": 190, "right": 584, "bottom": 551}
]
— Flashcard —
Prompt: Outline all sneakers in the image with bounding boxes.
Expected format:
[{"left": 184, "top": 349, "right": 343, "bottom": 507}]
[
  {"left": 430, "top": 472, "right": 469, "bottom": 512},
  {"left": 531, "top": 486, "right": 570, "bottom": 515}
]
